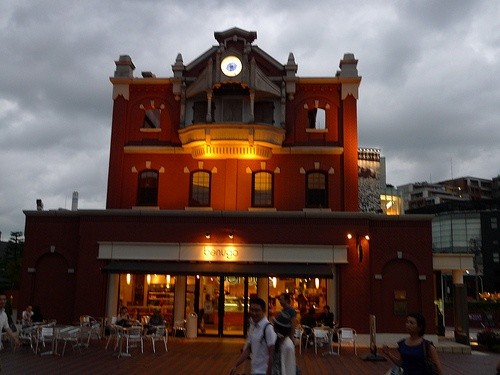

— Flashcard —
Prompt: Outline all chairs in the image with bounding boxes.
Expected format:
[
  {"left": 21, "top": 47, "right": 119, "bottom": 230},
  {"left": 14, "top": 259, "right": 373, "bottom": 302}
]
[
  {"left": 300, "top": 325, "right": 315, "bottom": 354},
  {"left": 13, "top": 316, "right": 169, "bottom": 359},
  {"left": 337, "top": 328, "right": 357, "bottom": 356}
]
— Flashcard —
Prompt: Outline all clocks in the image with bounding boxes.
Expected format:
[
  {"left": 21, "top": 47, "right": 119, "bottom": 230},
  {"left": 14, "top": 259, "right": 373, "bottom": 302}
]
[{"left": 219, "top": 54, "right": 243, "bottom": 77}]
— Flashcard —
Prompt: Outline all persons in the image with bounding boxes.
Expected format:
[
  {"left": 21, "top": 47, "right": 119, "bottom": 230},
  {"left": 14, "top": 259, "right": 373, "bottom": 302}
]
[
  {"left": 0, "top": 293, "right": 45, "bottom": 351},
  {"left": 318, "top": 305, "right": 340, "bottom": 353},
  {"left": 299, "top": 307, "right": 316, "bottom": 349},
  {"left": 273, "top": 292, "right": 301, "bottom": 375},
  {"left": 112, "top": 306, "right": 134, "bottom": 352},
  {"left": 130, "top": 306, "right": 165, "bottom": 348},
  {"left": 226, "top": 296, "right": 277, "bottom": 374},
  {"left": 270, "top": 307, "right": 297, "bottom": 375},
  {"left": 382, "top": 311, "right": 443, "bottom": 375}
]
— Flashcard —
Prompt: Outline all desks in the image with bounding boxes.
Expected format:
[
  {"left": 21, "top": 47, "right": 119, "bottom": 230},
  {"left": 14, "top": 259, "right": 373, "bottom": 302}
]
[
  {"left": 59, "top": 326, "right": 82, "bottom": 355},
  {"left": 313, "top": 327, "right": 329, "bottom": 343}
]
